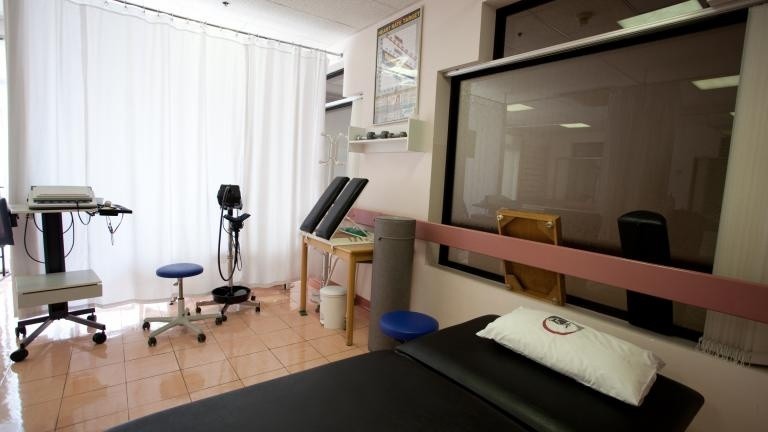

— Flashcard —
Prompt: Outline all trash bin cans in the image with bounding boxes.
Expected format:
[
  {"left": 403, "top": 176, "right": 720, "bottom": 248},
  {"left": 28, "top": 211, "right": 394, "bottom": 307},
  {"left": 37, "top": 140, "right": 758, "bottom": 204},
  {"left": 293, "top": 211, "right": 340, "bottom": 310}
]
[{"left": 318, "top": 285, "right": 347, "bottom": 330}]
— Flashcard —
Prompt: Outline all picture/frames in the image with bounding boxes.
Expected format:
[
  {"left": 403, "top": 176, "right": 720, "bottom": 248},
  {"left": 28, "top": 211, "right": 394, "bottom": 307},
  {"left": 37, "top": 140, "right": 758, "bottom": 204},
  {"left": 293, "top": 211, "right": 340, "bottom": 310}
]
[{"left": 369, "top": 5, "right": 424, "bottom": 126}]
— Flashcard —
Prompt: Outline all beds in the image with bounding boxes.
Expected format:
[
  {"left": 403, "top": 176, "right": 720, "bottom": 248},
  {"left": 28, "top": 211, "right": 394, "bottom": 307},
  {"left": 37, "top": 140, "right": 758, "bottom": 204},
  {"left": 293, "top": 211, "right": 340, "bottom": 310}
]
[{"left": 105, "top": 313, "right": 704, "bottom": 432}]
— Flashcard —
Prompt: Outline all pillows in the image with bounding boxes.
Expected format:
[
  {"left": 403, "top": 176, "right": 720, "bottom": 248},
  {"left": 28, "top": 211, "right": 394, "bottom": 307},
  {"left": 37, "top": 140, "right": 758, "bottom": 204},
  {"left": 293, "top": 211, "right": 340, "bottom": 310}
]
[{"left": 475, "top": 305, "right": 668, "bottom": 407}]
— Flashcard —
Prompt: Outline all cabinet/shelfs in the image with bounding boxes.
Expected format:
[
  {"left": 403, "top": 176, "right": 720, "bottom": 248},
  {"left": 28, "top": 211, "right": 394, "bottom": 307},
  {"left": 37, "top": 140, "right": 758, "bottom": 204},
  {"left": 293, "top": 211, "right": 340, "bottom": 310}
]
[{"left": 346, "top": 118, "right": 428, "bottom": 155}]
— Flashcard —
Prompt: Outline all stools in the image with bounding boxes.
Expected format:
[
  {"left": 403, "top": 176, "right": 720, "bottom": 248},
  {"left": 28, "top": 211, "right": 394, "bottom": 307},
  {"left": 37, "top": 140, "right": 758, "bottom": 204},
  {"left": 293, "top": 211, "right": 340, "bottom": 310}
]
[
  {"left": 379, "top": 309, "right": 439, "bottom": 345},
  {"left": 141, "top": 263, "right": 223, "bottom": 347}
]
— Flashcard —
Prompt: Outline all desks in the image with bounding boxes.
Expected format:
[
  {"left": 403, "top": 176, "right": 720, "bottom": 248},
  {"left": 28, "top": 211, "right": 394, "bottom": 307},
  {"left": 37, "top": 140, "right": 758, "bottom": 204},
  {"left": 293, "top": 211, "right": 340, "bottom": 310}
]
[{"left": 298, "top": 227, "right": 375, "bottom": 346}]
